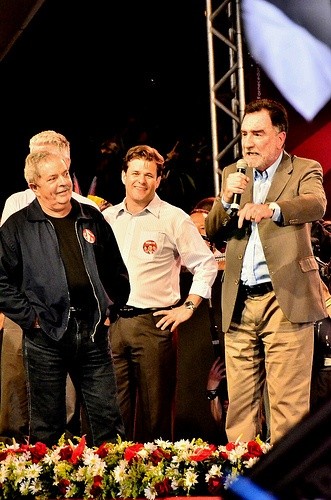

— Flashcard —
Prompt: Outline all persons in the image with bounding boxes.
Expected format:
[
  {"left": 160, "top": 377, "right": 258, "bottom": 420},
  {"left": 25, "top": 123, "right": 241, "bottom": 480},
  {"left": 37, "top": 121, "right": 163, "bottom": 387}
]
[
  {"left": 186, "top": 209, "right": 223, "bottom": 258},
  {"left": 0, "top": 130, "right": 101, "bottom": 446},
  {"left": 205, "top": 99, "right": 327, "bottom": 448},
  {"left": 206, "top": 357, "right": 226, "bottom": 422},
  {"left": 0, "top": 148, "right": 130, "bottom": 448},
  {"left": 101, "top": 145, "right": 218, "bottom": 446}
]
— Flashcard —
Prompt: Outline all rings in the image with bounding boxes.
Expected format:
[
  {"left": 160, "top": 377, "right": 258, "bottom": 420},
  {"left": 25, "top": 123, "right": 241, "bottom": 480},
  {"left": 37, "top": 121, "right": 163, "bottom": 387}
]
[{"left": 250, "top": 216, "right": 255, "bottom": 221}]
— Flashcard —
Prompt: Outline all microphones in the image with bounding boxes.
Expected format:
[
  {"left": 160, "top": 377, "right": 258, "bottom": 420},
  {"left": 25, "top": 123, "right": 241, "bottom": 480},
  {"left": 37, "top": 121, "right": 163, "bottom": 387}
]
[{"left": 231, "top": 159, "right": 248, "bottom": 212}]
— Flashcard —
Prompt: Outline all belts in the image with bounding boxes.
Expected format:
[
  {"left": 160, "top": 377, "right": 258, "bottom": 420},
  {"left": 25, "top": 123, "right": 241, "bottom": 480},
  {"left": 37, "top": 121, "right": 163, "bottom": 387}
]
[
  {"left": 115, "top": 306, "right": 174, "bottom": 319},
  {"left": 238, "top": 281, "right": 274, "bottom": 297}
]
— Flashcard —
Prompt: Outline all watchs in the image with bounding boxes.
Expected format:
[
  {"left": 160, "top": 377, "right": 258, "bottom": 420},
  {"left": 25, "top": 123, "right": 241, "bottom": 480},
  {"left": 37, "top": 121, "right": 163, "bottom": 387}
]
[
  {"left": 266, "top": 202, "right": 275, "bottom": 219},
  {"left": 184, "top": 301, "right": 196, "bottom": 310}
]
